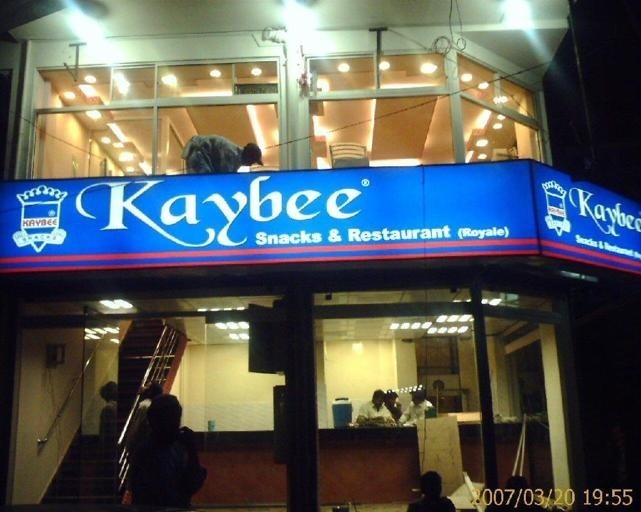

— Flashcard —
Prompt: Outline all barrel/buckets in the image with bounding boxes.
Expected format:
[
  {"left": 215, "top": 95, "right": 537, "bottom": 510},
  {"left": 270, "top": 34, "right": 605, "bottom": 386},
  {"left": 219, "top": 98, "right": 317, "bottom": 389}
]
[{"left": 332, "top": 397, "right": 354, "bottom": 428}]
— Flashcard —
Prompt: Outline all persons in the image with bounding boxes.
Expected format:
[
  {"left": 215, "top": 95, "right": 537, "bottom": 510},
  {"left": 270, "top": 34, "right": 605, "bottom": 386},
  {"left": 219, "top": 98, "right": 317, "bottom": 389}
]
[
  {"left": 383, "top": 391, "right": 404, "bottom": 421},
  {"left": 483, "top": 476, "right": 546, "bottom": 511},
  {"left": 180, "top": 134, "right": 264, "bottom": 175},
  {"left": 356, "top": 390, "right": 396, "bottom": 424},
  {"left": 123, "top": 380, "right": 162, "bottom": 457},
  {"left": 406, "top": 470, "right": 456, "bottom": 511},
  {"left": 398, "top": 386, "right": 436, "bottom": 426},
  {"left": 129, "top": 393, "right": 207, "bottom": 511},
  {"left": 98, "top": 381, "right": 118, "bottom": 494}
]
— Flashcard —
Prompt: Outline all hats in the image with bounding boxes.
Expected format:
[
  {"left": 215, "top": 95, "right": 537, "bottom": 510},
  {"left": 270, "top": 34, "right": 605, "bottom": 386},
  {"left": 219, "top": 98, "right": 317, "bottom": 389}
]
[{"left": 244, "top": 144, "right": 262, "bottom": 166}]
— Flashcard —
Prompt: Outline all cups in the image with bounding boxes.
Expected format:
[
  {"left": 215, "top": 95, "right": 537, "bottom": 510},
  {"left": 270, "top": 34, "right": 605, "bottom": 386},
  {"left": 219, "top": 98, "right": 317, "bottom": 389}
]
[{"left": 207, "top": 420, "right": 216, "bottom": 431}]
